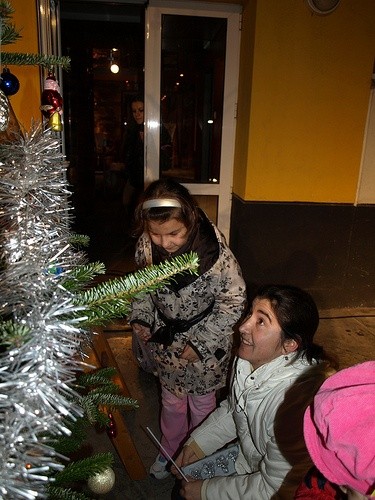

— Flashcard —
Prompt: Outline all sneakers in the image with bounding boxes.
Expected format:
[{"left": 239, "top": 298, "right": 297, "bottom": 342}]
[{"left": 149, "top": 452, "right": 172, "bottom": 480}]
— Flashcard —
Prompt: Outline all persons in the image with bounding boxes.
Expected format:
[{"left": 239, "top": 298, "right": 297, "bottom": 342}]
[
  {"left": 39, "top": 76, "right": 64, "bottom": 130},
  {"left": 170, "top": 283, "right": 338, "bottom": 500},
  {"left": 292, "top": 359, "right": 375, "bottom": 500},
  {"left": 128, "top": 176, "right": 249, "bottom": 481},
  {"left": 111, "top": 94, "right": 173, "bottom": 242}
]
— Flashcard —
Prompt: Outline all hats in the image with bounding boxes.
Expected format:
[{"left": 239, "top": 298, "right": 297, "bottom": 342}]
[{"left": 304, "top": 360, "right": 375, "bottom": 500}]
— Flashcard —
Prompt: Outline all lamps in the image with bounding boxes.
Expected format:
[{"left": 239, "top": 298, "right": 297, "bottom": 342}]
[{"left": 109, "top": 50, "right": 120, "bottom": 74}]
